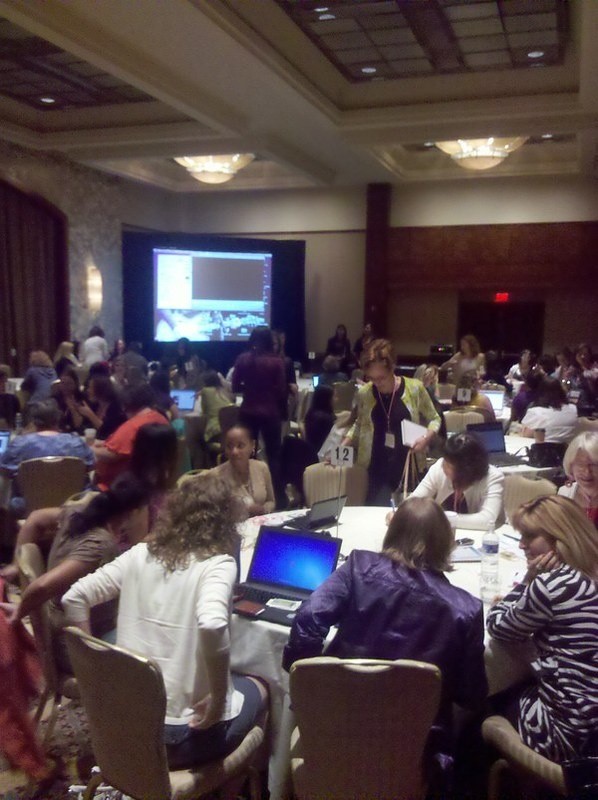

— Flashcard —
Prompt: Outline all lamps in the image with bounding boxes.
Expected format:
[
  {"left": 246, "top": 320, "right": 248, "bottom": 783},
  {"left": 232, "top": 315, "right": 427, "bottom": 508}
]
[
  {"left": 434, "top": 134, "right": 531, "bottom": 172},
  {"left": 174, "top": 151, "right": 257, "bottom": 183}
]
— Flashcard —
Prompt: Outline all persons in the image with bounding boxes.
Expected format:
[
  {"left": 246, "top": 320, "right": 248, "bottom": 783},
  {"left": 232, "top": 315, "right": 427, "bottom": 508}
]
[
  {"left": 0, "top": 322, "right": 598, "bottom": 800},
  {"left": 156, "top": 309, "right": 271, "bottom": 340}
]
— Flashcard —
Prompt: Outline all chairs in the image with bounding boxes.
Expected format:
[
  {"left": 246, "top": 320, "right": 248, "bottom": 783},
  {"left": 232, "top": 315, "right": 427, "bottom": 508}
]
[{"left": 0, "top": 364, "right": 598, "bottom": 800}]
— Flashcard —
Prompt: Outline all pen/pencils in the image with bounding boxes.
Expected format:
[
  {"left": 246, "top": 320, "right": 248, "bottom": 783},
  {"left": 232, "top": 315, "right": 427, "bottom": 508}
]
[
  {"left": 390, "top": 499, "right": 395, "bottom": 513},
  {"left": 503, "top": 534, "right": 520, "bottom": 541}
]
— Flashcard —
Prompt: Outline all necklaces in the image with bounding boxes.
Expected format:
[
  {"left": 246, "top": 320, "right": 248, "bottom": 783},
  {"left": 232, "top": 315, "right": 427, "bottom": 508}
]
[{"left": 244, "top": 484, "right": 249, "bottom": 487}]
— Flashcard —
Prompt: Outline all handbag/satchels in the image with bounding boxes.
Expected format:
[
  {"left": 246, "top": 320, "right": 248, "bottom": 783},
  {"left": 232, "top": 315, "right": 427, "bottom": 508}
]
[
  {"left": 528, "top": 441, "right": 569, "bottom": 467},
  {"left": 390, "top": 449, "right": 423, "bottom": 509}
]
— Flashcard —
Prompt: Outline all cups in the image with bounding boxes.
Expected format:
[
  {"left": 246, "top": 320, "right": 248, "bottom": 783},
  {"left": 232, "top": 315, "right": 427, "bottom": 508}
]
[{"left": 84, "top": 428, "right": 96, "bottom": 446}]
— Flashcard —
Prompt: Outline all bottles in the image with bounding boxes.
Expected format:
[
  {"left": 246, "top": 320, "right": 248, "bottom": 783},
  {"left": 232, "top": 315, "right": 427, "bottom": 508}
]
[
  {"left": 482, "top": 521, "right": 499, "bottom": 585},
  {"left": 14, "top": 413, "right": 23, "bottom": 434}
]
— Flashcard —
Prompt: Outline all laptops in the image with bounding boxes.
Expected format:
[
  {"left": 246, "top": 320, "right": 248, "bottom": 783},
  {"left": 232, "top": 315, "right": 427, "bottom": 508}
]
[
  {"left": 170, "top": 390, "right": 196, "bottom": 414},
  {"left": 479, "top": 390, "right": 504, "bottom": 416},
  {"left": 231, "top": 525, "right": 342, "bottom": 626},
  {"left": 0, "top": 430, "right": 10, "bottom": 463},
  {"left": 286, "top": 495, "right": 348, "bottom": 530},
  {"left": 467, "top": 421, "right": 526, "bottom": 468}
]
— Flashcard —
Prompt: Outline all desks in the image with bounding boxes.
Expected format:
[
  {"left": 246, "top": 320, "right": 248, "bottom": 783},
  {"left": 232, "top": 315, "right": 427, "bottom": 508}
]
[
  {"left": 442, "top": 397, "right": 512, "bottom": 435},
  {"left": 427, "top": 430, "right": 566, "bottom": 483},
  {"left": 228, "top": 504, "right": 541, "bottom": 800},
  {"left": 396, "top": 355, "right": 520, "bottom": 376},
  {"left": 165, "top": 406, "right": 205, "bottom": 447}
]
